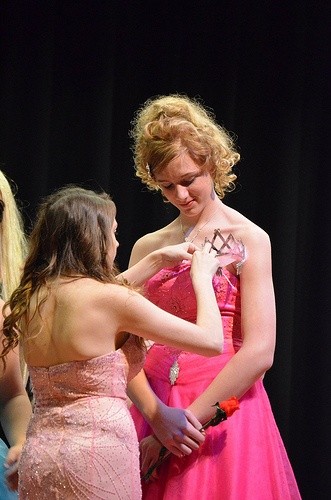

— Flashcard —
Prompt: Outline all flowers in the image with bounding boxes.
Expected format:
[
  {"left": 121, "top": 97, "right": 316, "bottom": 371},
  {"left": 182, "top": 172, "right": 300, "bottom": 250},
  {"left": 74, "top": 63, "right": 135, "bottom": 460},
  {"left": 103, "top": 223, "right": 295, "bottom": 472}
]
[{"left": 143, "top": 396, "right": 240, "bottom": 483}]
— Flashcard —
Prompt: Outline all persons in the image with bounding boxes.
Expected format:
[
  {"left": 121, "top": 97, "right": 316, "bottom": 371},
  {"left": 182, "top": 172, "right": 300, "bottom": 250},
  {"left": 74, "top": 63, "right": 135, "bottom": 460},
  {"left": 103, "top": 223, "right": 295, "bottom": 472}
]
[
  {"left": 123, "top": 93, "right": 302, "bottom": 500},
  {"left": 0, "top": 296, "right": 34, "bottom": 499},
  {"left": 0, "top": 168, "right": 50, "bottom": 405},
  {"left": 0, "top": 185, "right": 223, "bottom": 500}
]
